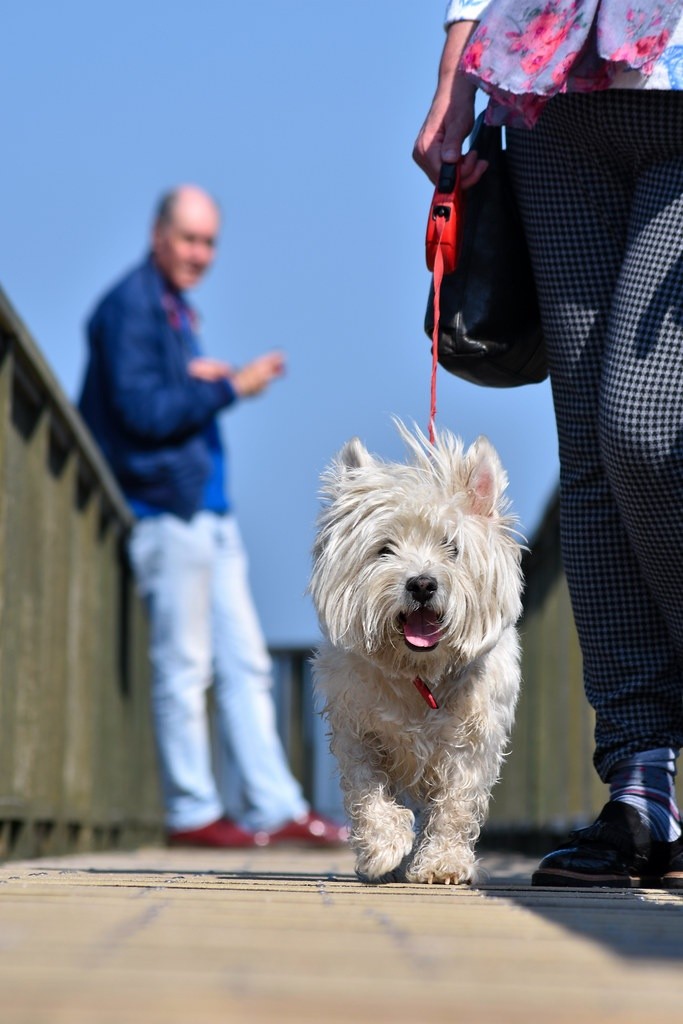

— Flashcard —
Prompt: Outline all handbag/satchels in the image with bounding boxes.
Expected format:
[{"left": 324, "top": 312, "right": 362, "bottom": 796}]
[{"left": 424, "top": 113, "right": 547, "bottom": 390}]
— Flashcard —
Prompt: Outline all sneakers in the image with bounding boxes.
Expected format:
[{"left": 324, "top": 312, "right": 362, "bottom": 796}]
[{"left": 530, "top": 801, "right": 683, "bottom": 894}]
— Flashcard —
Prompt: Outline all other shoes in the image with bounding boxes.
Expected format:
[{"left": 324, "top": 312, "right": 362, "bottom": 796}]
[{"left": 166, "top": 813, "right": 353, "bottom": 849}]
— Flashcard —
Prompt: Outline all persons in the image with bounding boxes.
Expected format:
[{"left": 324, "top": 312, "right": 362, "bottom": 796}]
[
  {"left": 75, "top": 186, "right": 358, "bottom": 849},
  {"left": 411, "top": 2, "right": 681, "bottom": 898}
]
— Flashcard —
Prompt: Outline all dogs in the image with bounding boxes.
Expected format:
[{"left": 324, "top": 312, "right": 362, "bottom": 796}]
[{"left": 301, "top": 412, "right": 532, "bottom": 885}]
